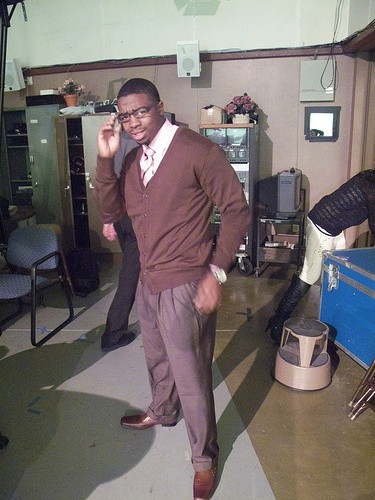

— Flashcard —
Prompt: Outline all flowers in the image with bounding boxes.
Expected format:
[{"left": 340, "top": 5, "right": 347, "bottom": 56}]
[
  {"left": 57, "top": 77, "right": 87, "bottom": 97},
  {"left": 222, "top": 91, "right": 258, "bottom": 116}
]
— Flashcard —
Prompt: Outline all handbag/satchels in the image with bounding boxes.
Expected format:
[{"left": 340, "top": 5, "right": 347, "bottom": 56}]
[{"left": 62, "top": 251, "right": 101, "bottom": 296}]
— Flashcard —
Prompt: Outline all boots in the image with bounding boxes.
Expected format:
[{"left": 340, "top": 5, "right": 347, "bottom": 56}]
[{"left": 264, "top": 272, "right": 312, "bottom": 342}]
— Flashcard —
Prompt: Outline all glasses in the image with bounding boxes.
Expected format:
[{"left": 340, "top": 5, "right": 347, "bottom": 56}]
[{"left": 117, "top": 101, "right": 160, "bottom": 124}]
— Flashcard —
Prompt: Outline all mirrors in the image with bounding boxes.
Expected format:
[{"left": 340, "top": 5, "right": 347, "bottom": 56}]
[{"left": 303, "top": 105, "right": 343, "bottom": 142}]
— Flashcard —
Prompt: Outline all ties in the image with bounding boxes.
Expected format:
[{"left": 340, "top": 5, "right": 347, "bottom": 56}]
[{"left": 140, "top": 147, "right": 155, "bottom": 188}]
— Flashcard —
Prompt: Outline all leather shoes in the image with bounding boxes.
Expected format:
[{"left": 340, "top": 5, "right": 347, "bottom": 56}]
[
  {"left": 193, "top": 467, "right": 217, "bottom": 500},
  {"left": 120, "top": 411, "right": 178, "bottom": 431},
  {"left": 102, "top": 332, "right": 137, "bottom": 352}
]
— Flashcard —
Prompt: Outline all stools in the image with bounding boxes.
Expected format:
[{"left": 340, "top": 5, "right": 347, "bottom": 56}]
[{"left": 279, "top": 317, "right": 330, "bottom": 369}]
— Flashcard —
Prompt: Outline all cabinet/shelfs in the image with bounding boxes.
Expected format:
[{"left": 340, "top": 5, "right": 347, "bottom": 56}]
[{"left": 0, "top": 103, "right": 174, "bottom": 256}]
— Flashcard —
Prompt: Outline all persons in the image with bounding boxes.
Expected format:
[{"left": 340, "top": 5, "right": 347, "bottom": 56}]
[
  {"left": 99, "top": 127, "right": 142, "bottom": 352},
  {"left": 265, "top": 169, "right": 375, "bottom": 346},
  {"left": 93, "top": 77, "right": 253, "bottom": 500}
]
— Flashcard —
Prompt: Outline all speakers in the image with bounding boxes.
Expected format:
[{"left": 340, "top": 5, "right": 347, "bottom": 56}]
[
  {"left": 177, "top": 40, "right": 201, "bottom": 78},
  {"left": 4, "top": 58, "right": 25, "bottom": 93}
]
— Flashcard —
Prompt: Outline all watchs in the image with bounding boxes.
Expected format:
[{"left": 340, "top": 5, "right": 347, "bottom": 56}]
[{"left": 211, "top": 266, "right": 228, "bottom": 287}]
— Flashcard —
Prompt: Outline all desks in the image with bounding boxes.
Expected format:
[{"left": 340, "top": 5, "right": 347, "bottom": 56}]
[{"left": 7, "top": 203, "right": 36, "bottom": 228}]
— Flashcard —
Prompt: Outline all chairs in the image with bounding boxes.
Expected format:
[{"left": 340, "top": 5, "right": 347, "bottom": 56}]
[{"left": 0, "top": 224, "right": 75, "bottom": 348}]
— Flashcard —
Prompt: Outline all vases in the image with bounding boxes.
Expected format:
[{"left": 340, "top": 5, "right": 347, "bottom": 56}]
[
  {"left": 231, "top": 114, "right": 250, "bottom": 124},
  {"left": 63, "top": 93, "right": 82, "bottom": 108}
]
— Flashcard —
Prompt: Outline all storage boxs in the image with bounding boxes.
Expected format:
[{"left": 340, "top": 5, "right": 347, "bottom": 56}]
[
  {"left": 319, "top": 245, "right": 375, "bottom": 372},
  {"left": 200, "top": 104, "right": 226, "bottom": 125}
]
